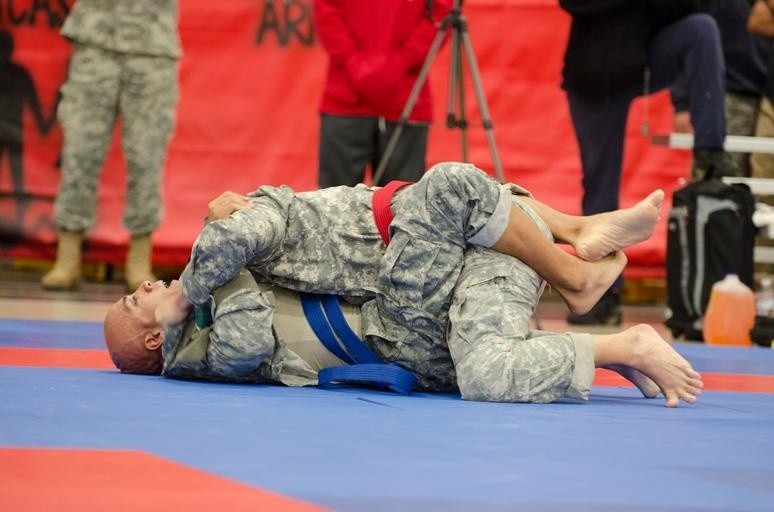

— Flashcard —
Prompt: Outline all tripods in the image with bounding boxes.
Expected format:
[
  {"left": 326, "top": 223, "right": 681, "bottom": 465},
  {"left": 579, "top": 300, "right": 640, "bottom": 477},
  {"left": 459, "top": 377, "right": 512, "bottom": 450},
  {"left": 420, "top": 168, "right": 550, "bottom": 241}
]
[{"left": 371, "top": 0, "right": 507, "bottom": 187}]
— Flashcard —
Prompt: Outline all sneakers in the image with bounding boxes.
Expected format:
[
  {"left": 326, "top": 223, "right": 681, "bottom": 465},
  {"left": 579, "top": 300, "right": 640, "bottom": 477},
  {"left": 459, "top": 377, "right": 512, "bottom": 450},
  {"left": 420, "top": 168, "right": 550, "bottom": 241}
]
[{"left": 568, "top": 289, "right": 626, "bottom": 325}]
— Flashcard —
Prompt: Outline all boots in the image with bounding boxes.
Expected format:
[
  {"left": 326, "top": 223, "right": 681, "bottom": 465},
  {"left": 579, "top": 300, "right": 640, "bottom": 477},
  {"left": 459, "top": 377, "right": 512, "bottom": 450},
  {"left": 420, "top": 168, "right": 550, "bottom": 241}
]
[
  {"left": 40, "top": 230, "right": 87, "bottom": 288},
  {"left": 124, "top": 232, "right": 159, "bottom": 293}
]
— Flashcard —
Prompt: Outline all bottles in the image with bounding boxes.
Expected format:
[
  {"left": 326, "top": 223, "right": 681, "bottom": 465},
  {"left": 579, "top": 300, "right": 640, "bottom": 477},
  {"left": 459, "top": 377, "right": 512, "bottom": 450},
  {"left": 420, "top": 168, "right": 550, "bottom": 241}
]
[{"left": 755, "top": 275, "right": 774, "bottom": 318}]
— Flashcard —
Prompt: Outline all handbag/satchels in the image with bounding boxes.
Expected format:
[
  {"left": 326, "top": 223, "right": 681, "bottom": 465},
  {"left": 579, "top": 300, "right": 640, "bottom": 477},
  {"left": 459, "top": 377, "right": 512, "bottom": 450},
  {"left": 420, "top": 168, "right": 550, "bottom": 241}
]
[{"left": 663, "top": 176, "right": 756, "bottom": 343}]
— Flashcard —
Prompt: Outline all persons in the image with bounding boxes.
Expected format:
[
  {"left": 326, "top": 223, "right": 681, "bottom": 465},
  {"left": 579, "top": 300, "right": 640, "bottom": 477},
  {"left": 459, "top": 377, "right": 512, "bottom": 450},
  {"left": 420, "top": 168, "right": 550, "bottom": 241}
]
[
  {"left": 670, "top": 0, "right": 774, "bottom": 181},
  {"left": 311, "top": 0, "right": 463, "bottom": 188},
  {"left": 102, "top": 159, "right": 665, "bottom": 393},
  {"left": 39, "top": 2, "right": 182, "bottom": 291},
  {"left": 742, "top": 0, "right": 774, "bottom": 347},
  {"left": 151, "top": 177, "right": 705, "bottom": 408},
  {"left": 555, "top": 0, "right": 729, "bottom": 327}
]
